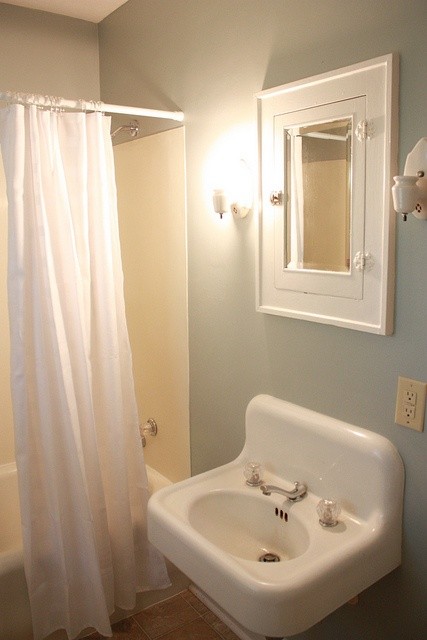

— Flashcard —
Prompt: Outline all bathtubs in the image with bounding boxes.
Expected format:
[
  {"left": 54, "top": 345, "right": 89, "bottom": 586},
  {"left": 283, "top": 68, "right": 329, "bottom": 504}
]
[{"left": 1, "top": 463, "right": 184, "bottom": 640}]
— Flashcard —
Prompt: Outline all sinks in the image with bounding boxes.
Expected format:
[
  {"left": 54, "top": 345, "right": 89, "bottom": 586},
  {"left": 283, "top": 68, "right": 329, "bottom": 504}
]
[{"left": 148, "top": 462, "right": 381, "bottom": 637}]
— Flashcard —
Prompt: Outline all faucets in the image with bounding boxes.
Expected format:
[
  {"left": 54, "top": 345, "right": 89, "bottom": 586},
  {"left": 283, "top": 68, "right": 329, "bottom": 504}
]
[{"left": 260, "top": 481, "right": 306, "bottom": 500}]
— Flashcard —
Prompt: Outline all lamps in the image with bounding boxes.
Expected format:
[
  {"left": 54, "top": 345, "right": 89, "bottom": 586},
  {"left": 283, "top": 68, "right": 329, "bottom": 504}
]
[
  {"left": 204, "top": 157, "right": 248, "bottom": 218},
  {"left": 392, "top": 149, "right": 427, "bottom": 223}
]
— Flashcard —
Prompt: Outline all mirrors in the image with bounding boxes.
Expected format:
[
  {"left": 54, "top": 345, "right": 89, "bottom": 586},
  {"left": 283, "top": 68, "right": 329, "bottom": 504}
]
[{"left": 250, "top": 53, "right": 397, "bottom": 336}]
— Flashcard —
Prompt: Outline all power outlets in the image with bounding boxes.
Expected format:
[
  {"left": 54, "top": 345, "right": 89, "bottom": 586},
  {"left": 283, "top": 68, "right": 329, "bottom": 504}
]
[{"left": 393, "top": 376, "right": 427, "bottom": 435}]
